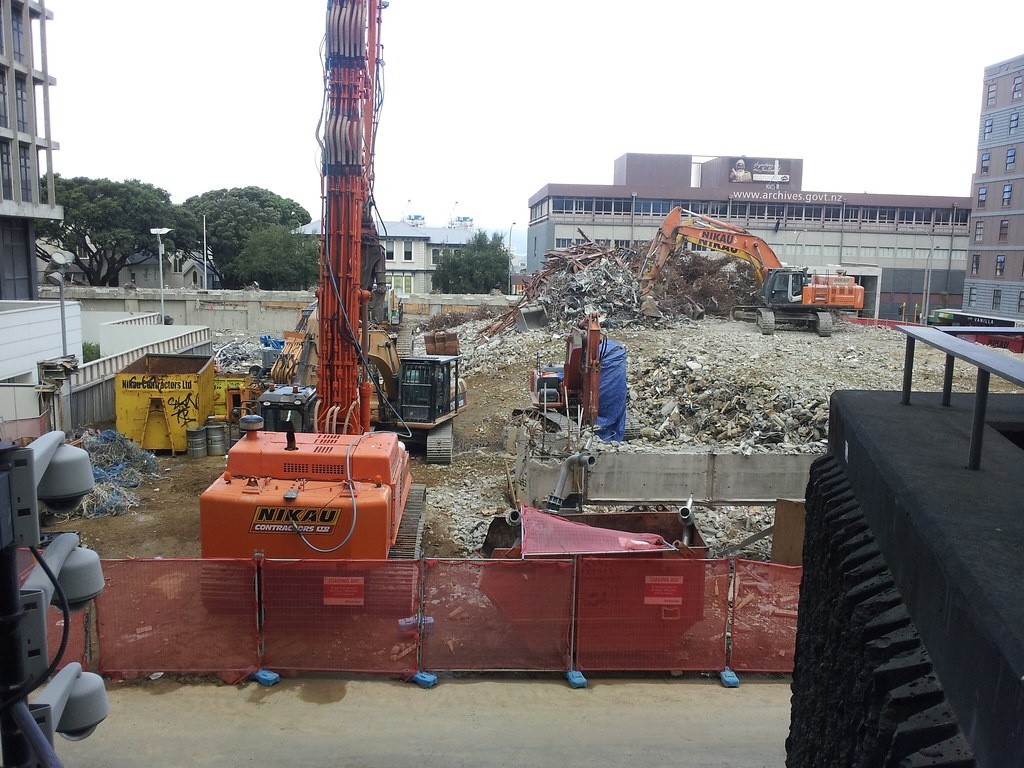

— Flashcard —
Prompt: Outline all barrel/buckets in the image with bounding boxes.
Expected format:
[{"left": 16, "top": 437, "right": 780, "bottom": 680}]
[
  {"left": 186, "top": 428, "right": 207, "bottom": 457},
  {"left": 206, "top": 426, "right": 226, "bottom": 456}
]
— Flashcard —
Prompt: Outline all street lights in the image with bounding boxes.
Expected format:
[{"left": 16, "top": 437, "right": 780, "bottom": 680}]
[
  {"left": 151, "top": 227, "right": 172, "bottom": 324},
  {"left": 920, "top": 246, "right": 941, "bottom": 322},
  {"left": 43, "top": 250, "right": 75, "bottom": 356},
  {"left": 909, "top": 226, "right": 934, "bottom": 324}
]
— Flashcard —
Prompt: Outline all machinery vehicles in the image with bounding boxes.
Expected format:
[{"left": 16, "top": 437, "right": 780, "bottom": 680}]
[
  {"left": 198, "top": 1, "right": 431, "bottom": 618},
  {"left": 640, "top": 205, "right": 864, "bottom": 337},
  {"left": 257, "top": 322, "right": 469, "bottom": 465}
]
